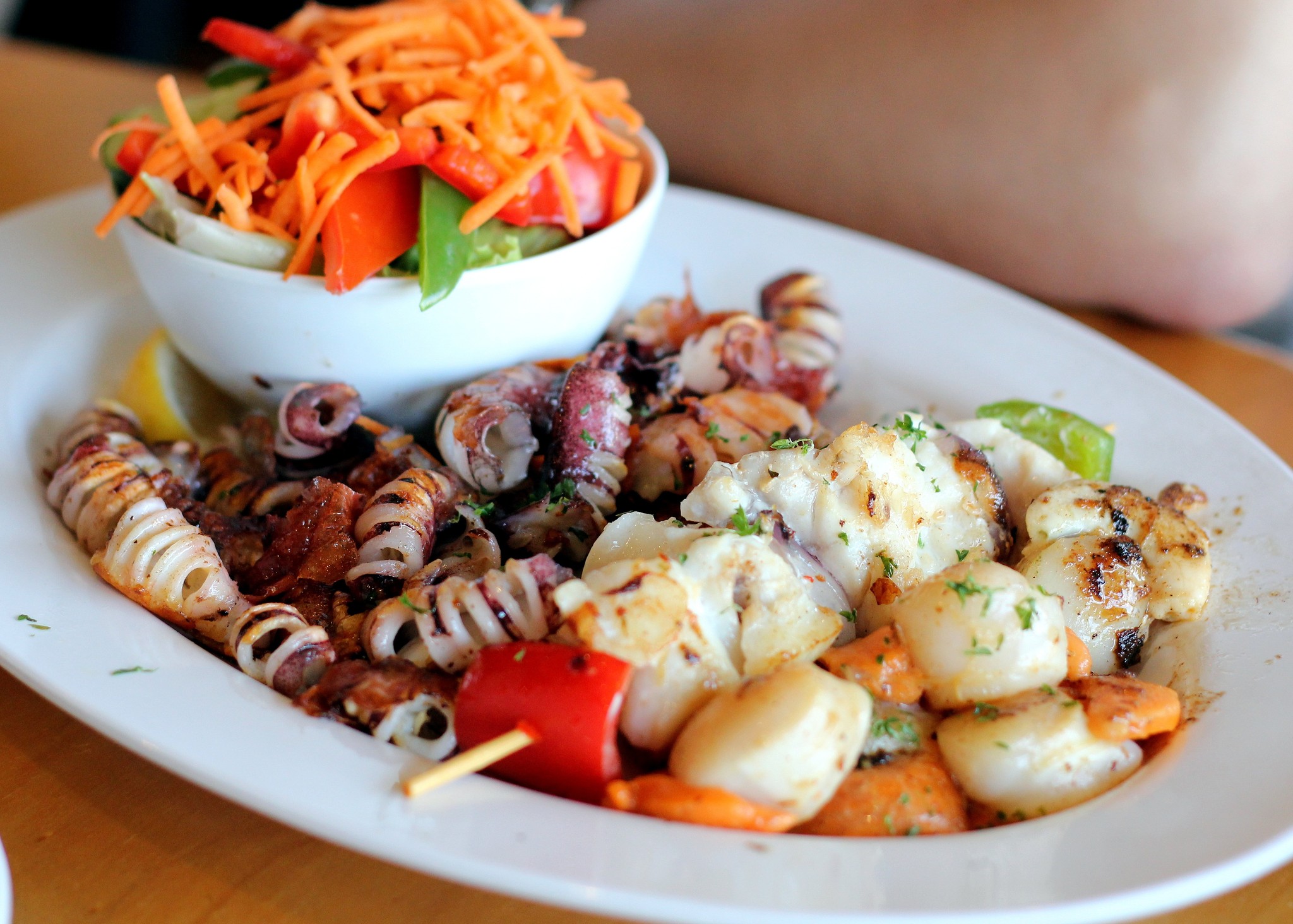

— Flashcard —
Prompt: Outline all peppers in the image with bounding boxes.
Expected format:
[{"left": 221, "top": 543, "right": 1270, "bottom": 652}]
[
  {"left": 448, "top": 650, "right": 637, "bottom": 822},
  {"left": 86, "top": 13, "right": 642, "bottom": 311},
  {"left": 976, "top": 392, "right": 1113, "bottom": 483}
]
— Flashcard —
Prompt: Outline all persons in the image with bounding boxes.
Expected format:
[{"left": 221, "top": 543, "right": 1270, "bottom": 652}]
[{"left": 516, "top": 1, "right": 1293, "bottom": 360}]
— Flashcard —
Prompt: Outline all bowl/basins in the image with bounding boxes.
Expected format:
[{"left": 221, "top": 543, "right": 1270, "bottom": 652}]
[{"left": 105, "top": 122, "right": 668, "bottom": 432}]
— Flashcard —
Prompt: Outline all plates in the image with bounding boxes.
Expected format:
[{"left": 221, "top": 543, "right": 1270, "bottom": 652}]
[{"left": 0, "top": 176, "right": 1293, "bottom": 923}]
